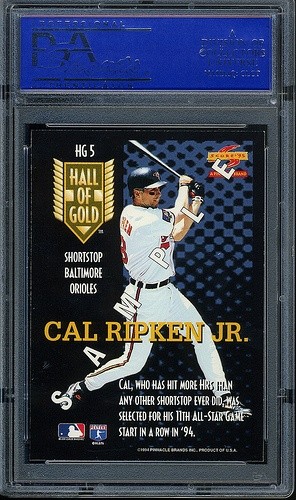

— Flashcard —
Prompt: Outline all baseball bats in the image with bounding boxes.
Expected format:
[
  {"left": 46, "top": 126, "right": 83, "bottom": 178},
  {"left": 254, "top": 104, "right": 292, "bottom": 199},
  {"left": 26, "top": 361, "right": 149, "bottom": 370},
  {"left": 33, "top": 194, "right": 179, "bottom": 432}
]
[{"left": 130, "top": 140, "right": 201, "bottom": 189}]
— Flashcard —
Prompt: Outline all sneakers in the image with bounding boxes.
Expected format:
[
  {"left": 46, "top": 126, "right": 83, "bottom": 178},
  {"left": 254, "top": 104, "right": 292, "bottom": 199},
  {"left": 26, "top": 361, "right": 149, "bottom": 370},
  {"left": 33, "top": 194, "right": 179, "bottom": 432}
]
[
  {"left": 60, "top": 382, "right": 85, "bottom": 411},
  {"left": 218, "top": 400, "right": 253, "bottom": 421}
]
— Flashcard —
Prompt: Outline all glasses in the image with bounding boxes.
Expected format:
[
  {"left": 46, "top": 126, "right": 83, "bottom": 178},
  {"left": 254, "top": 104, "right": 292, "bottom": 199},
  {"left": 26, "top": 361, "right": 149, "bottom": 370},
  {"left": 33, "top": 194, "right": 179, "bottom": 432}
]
[{"left": 145, "top": 189, "right": 158, "bottom": 195}]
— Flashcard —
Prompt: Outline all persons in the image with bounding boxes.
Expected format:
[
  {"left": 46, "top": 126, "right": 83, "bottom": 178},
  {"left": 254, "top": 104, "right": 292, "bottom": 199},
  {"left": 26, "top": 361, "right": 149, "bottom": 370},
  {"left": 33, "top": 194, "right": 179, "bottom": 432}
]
[{"left": 59, "top": 166, "right": 253, "bottom": 417}]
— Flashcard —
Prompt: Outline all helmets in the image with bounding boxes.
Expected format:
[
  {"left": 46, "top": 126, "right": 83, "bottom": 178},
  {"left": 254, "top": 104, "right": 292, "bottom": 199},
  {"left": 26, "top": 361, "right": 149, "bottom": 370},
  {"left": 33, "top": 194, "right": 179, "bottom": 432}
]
[{"left": 128, "top": 166, "right": 168, "bottom": 198}]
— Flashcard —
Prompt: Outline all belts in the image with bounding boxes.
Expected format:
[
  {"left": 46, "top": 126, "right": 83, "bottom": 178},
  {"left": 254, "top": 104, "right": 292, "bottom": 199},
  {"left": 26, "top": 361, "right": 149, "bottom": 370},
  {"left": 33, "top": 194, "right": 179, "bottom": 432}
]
[{"left": 130, "top": 279, "right": 170, "bottom": 289}]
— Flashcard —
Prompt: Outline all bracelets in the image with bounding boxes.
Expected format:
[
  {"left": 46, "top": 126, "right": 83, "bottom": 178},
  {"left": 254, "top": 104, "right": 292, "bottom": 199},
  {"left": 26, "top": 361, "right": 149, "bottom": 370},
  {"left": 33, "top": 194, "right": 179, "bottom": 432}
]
[{"left": 179, "top": 183, "right": 189, "bottom": 187}]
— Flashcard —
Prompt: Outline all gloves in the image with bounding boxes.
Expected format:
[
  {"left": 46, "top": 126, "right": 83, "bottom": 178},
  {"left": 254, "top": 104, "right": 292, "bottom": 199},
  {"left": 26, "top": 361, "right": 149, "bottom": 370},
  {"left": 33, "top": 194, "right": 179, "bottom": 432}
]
[{"left": 192, "top": 182, "right": 205, "bottom": 202}]
